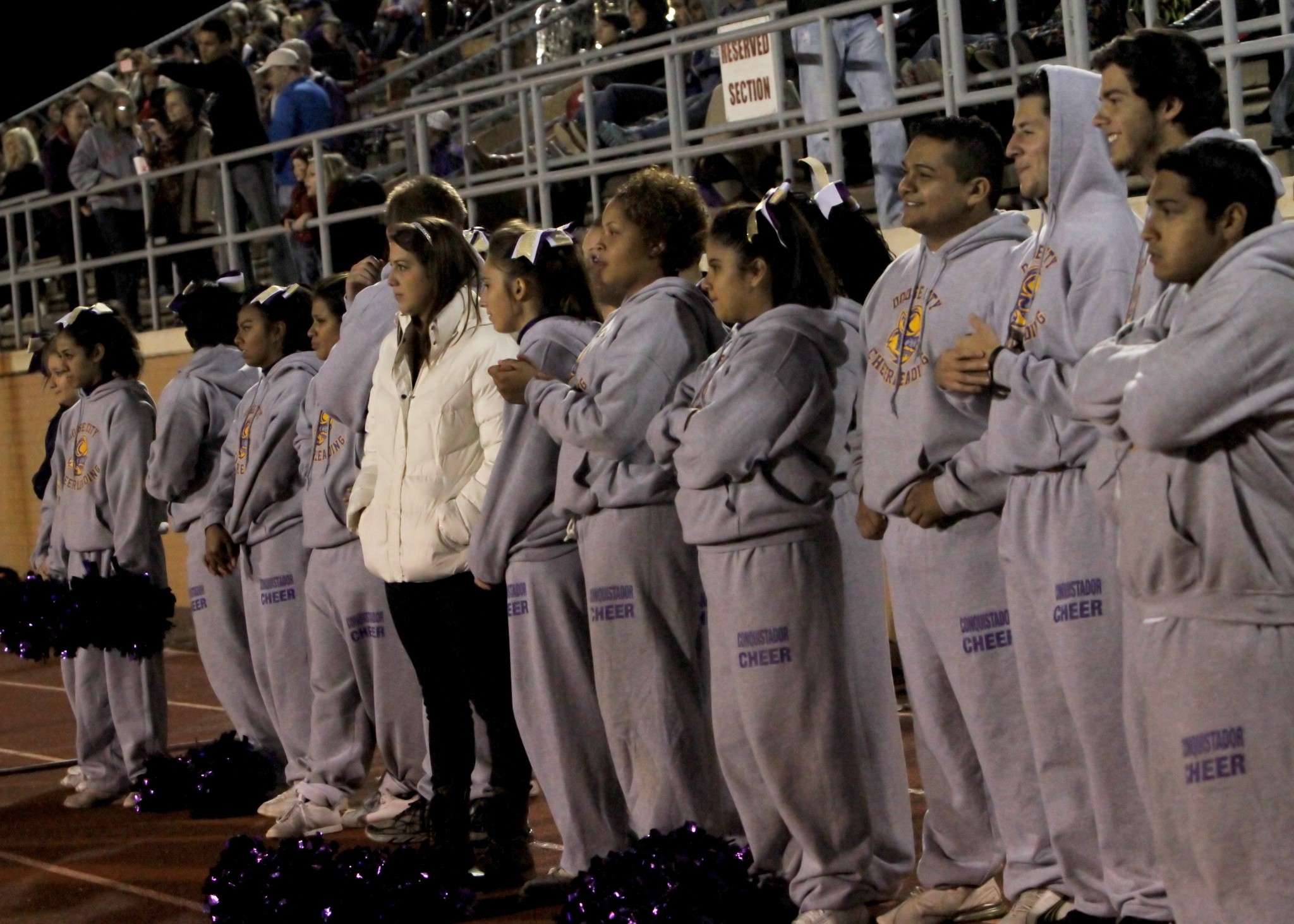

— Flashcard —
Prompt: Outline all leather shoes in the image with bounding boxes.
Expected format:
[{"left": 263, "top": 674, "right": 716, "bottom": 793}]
[{"left": 468, "top": 139, "right": 502, "bottom": 172}]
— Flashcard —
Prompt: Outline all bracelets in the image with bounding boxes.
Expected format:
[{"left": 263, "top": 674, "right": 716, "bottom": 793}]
[{"left": 987, "top": 345, "right": 1011, "bottom": 394}]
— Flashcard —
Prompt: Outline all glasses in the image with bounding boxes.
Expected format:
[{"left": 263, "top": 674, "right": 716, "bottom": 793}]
[{"left": 117, "top": 105, "right": 139, "bottom": 115}]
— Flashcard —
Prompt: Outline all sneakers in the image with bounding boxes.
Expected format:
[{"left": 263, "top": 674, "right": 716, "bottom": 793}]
[
  {"left": 122, "top": 792, "right": 142, "bottom": 807},
  {"left": 74, "top": 779, "right": 90, "bottom": 793},
  {"left": 876, "top": 876, "right": 1009, "bottom": 924},
  {"left": 598, "top": 120, "right": 628, "bottom": 148},
  {"left": 366, "top": 796, "right": 437, "bottom": 842},
  {"left": 61, "top": 764, "right": 84, "bottom": 792},
  {"left": 340, "top": 792, "right": 422, "bottom": 828},
  {"left": 266, "top": 800, "right": 344, "bottom": 840},
  {"left": 258, "top": 787, "right": 307, "bottom": 817},
  {"left": 64, "top": 784, "right": 113, "bottom": 809},
  {"left": 998, "top": 887, "right": 1064, "bottom": 924},
  {"left": 792, "top": 904, "right": 873, "bottom": 924},
  {"left": 521, "top": 864, "right": 577, "bottom": 901}
]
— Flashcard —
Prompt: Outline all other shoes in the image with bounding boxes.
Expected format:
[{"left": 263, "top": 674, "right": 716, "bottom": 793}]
[
  {"left": 467, "top": 845, "right": 535, "bottom": 892},
  {"left": 898, "top": 9, "right": 1180, "bottom": 87}
]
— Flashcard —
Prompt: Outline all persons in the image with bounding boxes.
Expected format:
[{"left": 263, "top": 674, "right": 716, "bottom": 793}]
[
  {"left": 0, "top": 0, "right": 1294, "bottom": 356},
  {"left": 1070, "top": 134, "right": 1293, "bottom": 924},
  {"left": 1086, "top": 26, "right": 1287, "bottom": 527},
  {"left": 645, "top": 179, "right": 883, "bottom": 924},
  {"left": 488, "top": 161, "right": 752, "bottom": 856},
  {"left": 843, "top": 114, "right": 1077, "bottom": 923},
  {"left": 460, "top": 176, "right": 734, "bottom": 335},
  {"left": 28, "top": 330, "right": 94, "bottom": 793},
  {"left": 199, "top": 282, "right": 327, "bottom": 818},
  {"left": 928, "top": 64, "right": 1177, "bottom": 923},
  {"left": 308, "top": 174, "right": 497, "bottom": 844},
  {"left": 28, "top": 303, "right": 169, "bottom": 812},
  {"left": 343, "top": 216, "right": 538, "bottom": 892},
  {"left": 141, "top": 268, "right": 288, "bottom": 770},
  {"left": 788, "top": 155, "right": 919, "bottom": 904},
  {"left": 260, "top": 272, "right": 428, "bottom": 840}
]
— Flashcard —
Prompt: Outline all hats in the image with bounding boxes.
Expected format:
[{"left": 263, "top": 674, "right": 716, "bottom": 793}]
[
  {"left": 91, "top": 72, "right": 117, "bottom": 94},
  {"left": 252, "top": 48, "right": 301, "bottom": 74},
  {"left": 426, "top": 109, "right": 453, "bottom": 132},
  {"left": 601, "top": 12, "right": 629, "bottom": 32}
]
